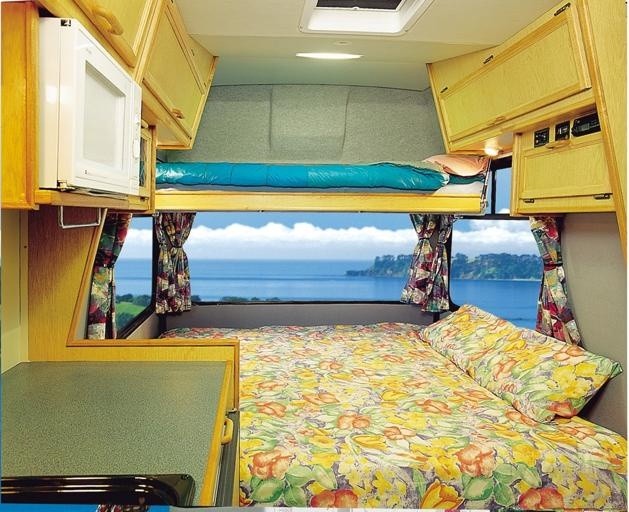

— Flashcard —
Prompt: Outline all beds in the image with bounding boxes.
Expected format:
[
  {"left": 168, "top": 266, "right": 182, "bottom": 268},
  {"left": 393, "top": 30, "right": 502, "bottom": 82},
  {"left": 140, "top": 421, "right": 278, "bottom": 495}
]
[
  {"left": 156, "top": 322, "right": 627, "bottom": 510},
  {"left": 143, "top": 163, "right": 491, "bottom": 215}
]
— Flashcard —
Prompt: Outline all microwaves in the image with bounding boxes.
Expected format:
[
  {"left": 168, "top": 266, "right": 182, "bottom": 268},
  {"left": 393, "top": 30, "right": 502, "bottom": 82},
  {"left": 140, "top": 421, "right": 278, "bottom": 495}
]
[{"left": 42, "top": 11, "right": 140, "bottom": 197}]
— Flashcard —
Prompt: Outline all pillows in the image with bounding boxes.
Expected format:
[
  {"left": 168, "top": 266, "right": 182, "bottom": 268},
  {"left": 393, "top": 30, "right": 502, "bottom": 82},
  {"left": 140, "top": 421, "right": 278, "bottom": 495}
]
[
  {"left": 417, "top": 302, "right": 621, "bottom": 428},
  {"left": 421, "top": 154, "right": 484, "bottom": 177}
]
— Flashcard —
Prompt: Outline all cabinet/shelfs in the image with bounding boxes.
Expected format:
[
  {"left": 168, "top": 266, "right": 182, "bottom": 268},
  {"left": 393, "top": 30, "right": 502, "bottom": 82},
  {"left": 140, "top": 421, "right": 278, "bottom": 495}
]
[
  {"left": 0, "top": 0, "right": 217, "bottom": 212},
  {"left": 424, "top": 1, "right": 625, "bottom": 214}
]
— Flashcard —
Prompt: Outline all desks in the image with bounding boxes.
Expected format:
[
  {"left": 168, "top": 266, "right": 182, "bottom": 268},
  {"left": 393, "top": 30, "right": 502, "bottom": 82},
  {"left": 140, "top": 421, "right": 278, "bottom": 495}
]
[{"left": 2, "top": 361, "right": 243, "bottom": 509}]
